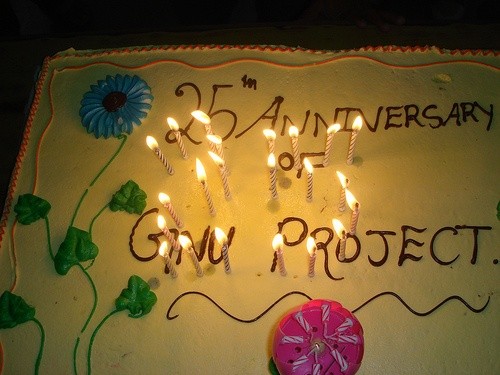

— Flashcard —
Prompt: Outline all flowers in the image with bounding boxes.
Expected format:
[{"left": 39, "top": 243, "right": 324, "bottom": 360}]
[{"left": 0, "top": 70, "right": 157, "bottom": 375}]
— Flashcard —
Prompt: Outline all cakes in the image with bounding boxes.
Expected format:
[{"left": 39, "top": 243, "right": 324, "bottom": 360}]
[{"left": 0, "top": 42, "right": 500, "bottom": 375}]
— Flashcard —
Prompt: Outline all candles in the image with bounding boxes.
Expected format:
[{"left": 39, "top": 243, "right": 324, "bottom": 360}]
[
  {"left": 322, "top": 340, "right": 349, "bottom": 371},
  {"left": 281, "top": 335, "right": 309, "bottom": 344},
  {"left": 336, "top": 169, "right": 349, "bottom": 212},
  {"left": 195, "top": 157, "right": 217, "bottom": 218},
  {"left": 215, "top": 226, "right": 232, "bottom": 274},
  {"left": 157, "top": 241, "right": 179, "bottom": 278},
  {"left": 266, "top": 152, "right": 278, "bottom": 200},
  {"left": 346, "top": 115, "right": 363, "bottom": 165},
  {"left": 302, "top": 157, "right": 314, "bottom": 203},
  {"left": 292, "top": 346, "right": 317, "bottom": 372},
  {"left": 344, "top": 187, "right": 361, "bottom": 235},
  {"left": 272, "top": 233, "right": 288, "bottom": 276},
  {"left": 158, "top": 191, "right": 185, "bottom": 229},
  {"left": 324, "top": 334, "right": 359, "bottom": 343},
  {"left": 332, "top": 217, "right": 347, "bottom": 262},
  {"left": 190, "top": 109, "right": 217, "bottom": 155},
  {"left": 320, "top": 304, "right": 332, "bottom": 339},
  {"left": 293, "top": 312, "right": 315, "bottom": 344},
  {"left": 157, "top": 215, "right": 181, "bottom": 252},
  {"left": 178, "top": 233, "right": 204, "bottom": 277},
  {"left": 208, "top": 151, "right": 232, "bottom": 201},
  {"left": 262, "top": 128, "right": 276, "bottom": 178},
  {"left": 166, "top": 117, "right": 191, "bottom": 161},
  {"left": 306, "top": 236, "right": 317, "bottom": 279},
  {"left": 321, "top": 123, "right": 341, "bottom": 167},
  {"left": 146, "top": 136, "right": 175, "bottom": 176},
  {"left": 325, "top": 317, "right": 354, "bottom": 337},
  {"left": 312, "top": 350, "right": 321, "bottom": 375},
  {"left": 205, "top": 135, "right": 232, "bottom": 175},
  {"left": 288, "top": 125, "right": 302, "bottom": 168}
]
